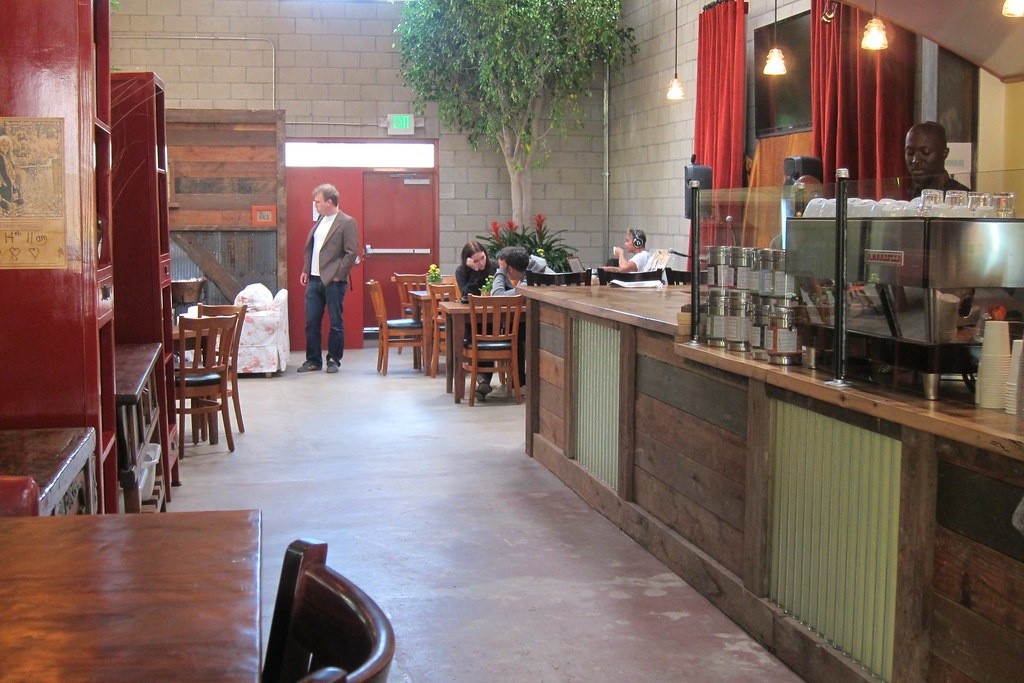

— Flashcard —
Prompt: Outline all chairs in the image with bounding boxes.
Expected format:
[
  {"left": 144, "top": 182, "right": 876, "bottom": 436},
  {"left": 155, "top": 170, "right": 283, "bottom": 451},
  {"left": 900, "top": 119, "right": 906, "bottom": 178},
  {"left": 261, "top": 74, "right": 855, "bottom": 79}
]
[
  {"left": 665, "top": 266, "right": 707, "bottom": 286},
  {"left": 175, "top": 304, "right": 250, "bottom": 462},
  {"left": 525, "top": 268, "right": 592, "bottom": 287},
  {"left": 259, "top": 534, "right": 398, "bottom": 683},
  {"left": 182, "top": 282, "right": 290, "bottom": 379},
  {"left": 368, "top": 271, "right": 523, "bottom": 406},
  {"left": 598, "top": 265, "right": 663, "bottom": 287}
]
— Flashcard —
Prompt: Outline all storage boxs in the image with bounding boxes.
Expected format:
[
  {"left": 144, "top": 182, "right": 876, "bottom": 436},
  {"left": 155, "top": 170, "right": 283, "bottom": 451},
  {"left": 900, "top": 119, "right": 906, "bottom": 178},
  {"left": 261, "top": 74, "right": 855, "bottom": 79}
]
[
  {"left": 143, "top": 444, "right": 161, "bottom": 500},
  {"left": 118, "top": 467, "right": 150, "bottom": 515}
]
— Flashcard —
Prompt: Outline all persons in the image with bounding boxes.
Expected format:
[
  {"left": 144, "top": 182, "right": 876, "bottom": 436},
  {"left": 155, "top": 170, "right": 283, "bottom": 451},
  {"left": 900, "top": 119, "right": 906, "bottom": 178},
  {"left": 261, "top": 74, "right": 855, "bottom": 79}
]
[
  {"left": 770, "top": 174, "right": 824, "bottom": 249},
  {"left": 877, "top": 121, "right": 989, "bottom": 317},
  {"left": 599, "top": 228, "right": 649, "bottom": 273},
  {"left": 455, "top": 240, "right": 557, "bottom": 400},
  {"left": 297, "top": 184, "right": 358, "bottom": 373}
]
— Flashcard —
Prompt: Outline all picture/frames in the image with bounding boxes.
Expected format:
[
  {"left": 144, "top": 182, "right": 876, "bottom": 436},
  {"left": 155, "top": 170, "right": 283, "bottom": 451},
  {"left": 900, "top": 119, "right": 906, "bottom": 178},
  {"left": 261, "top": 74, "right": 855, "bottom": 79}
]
[{"left": 251, "top": 204, "right": 277, "bottom": 226}]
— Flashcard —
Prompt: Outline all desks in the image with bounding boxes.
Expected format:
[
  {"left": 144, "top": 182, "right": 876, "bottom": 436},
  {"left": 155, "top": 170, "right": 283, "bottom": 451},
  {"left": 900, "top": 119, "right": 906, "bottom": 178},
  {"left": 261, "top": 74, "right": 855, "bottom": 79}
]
[
  {"left": 438, "top": 300, "right": 525, "bottom": 405},
  {"left": 112, "top": 342, "right": 171, "bottom": 515},
  {"left": 0, "top": 506, "right": 265, "bottom": 683},
  {"left": 406, "top": 290, "right": 450, "bottom": 374}
]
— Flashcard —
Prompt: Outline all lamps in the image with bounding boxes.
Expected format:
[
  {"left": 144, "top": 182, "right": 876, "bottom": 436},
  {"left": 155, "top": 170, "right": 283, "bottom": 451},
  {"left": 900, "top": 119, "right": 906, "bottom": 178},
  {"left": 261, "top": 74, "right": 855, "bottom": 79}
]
[
  {"left": 1001, "top": 0, "right": 1024, "bottom": 18},
  {"left": 378, "top": 113, "right": 425, "bottom": 135},
  {"left": 860, "top": 0, "right": 888, "bottom": 51},
  {"left": 666, "top": 0, "right": 687, "bottom": 100},
  {"left": 762, "top": 0, "right": 787, "bottom": 75}
]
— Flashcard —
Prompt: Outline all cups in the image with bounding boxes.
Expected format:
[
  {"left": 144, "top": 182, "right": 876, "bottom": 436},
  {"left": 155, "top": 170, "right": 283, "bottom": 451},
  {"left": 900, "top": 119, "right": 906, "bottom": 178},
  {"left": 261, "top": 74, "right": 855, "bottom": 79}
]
[
  {"left": 803, "top": 189, "right": 1016, "bottom": 219},
  {"left": 972, "top": 312, "right": 992, "bottom": 344},
  {"left": 706, "top": 244, "right": 796, "bottom": 365},
  {"left": 974, "top": 321, "right": 1024, "bottom": 415},
  {"left": 925, "top": 291, "right": 960, "bottom": 342}
]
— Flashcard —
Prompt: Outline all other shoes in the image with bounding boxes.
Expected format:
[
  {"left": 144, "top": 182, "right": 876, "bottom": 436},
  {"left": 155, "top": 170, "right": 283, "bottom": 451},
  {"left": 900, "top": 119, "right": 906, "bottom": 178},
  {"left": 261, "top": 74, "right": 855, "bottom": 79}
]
[{"left": 474, "top": 380, "right": 492, "bottom": 402}]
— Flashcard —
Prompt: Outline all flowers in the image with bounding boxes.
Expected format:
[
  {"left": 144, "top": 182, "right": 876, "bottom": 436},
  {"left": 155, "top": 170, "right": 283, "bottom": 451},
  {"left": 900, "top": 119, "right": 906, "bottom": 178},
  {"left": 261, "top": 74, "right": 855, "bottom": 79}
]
[{"left": 425, "top": 212, "right": 582, "bottom": 294}]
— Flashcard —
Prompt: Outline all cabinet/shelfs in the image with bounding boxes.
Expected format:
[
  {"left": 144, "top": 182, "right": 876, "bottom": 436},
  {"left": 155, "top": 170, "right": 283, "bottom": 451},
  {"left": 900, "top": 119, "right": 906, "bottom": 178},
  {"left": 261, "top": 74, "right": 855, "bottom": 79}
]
[{"left": 0, "top": 0, "right": 179, "bottom": 516}]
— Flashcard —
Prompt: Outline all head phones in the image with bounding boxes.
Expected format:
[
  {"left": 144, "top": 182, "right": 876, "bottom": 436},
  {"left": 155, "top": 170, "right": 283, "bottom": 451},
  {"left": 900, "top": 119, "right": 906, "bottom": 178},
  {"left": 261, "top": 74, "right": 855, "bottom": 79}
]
[{"left": 632, "top": 230, "right": 643, "bottom": 249}]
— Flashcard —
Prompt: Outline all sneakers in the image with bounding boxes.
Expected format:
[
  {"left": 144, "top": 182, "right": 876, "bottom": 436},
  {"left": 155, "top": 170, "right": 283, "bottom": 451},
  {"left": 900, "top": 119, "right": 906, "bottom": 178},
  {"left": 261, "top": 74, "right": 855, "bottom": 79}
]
[
  {"left": 327, "top": 360, "right": 338, "bottom": 373},
  {"left": 487, "top": 383, "right": 526, "bottom": 399},
  {"left": 298, "top": 361, "right": 322, "bottom": 372}
]
